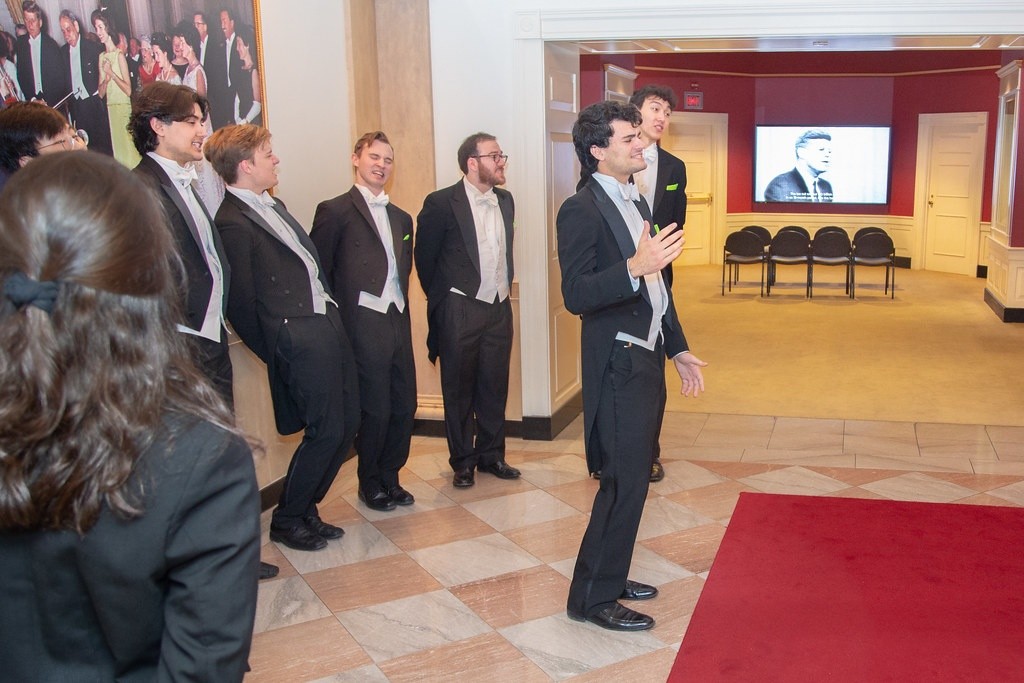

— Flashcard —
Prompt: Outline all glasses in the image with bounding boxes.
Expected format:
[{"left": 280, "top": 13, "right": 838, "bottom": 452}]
[
  {"left": 139, "top": 47, "right": 151, "bottom": 52},
  {"left": 24, "top": 129, "right": 89, "bottom": 155},
  {"left": 470, "top": 153, "right": 509, "bottom": 164}
]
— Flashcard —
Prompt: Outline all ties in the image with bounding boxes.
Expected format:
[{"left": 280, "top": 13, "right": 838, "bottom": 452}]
[{"left": 813, "top": 181, "right": 820, "bottom": 202}]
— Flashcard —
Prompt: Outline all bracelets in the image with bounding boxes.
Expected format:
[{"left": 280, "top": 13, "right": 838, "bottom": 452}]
[
  {"left": 103, "top": 80, "right": 108, "bottom": 83},
  {"left": 112, "top": 75, "right": 115, "bottom": 79}
]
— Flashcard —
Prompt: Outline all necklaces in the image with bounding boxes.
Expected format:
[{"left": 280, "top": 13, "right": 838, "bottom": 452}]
[{"left": 162, "top": 64, "right": 172, "bottom": 81}]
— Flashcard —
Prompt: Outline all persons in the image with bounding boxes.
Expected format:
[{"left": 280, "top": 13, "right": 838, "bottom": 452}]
[
  {"left": 311, "top": 130, "right": 416, "bottom": 512},
  {"left": 128, "top": 80, "right": 280, "bottom": 580},
  {"left": 575, "top": 85, "right": 689, "bottom": 483},
  {"left": 0, "top": 101, "right": 90, "bottom": 177},
  {"left": 0, "top": 0, "right": 262, "bottom": 171},
  {"left": 556, "top": 101, "right": 708, "bottom": 632},
  {"left": 202, "top": 124, "right": 364, "bottom": 551},
  {"left": 763, "top": 131, "right": 833, "bottom": 203},
  {"left": 0, "top": 151, "right": 260, "bottom": 683},
  {"left": 414, "top": 133, "right": 522, "bottom": 489}
]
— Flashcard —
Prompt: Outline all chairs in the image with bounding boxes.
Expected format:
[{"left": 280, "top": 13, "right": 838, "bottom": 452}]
[{"left": 722, "top": 226, "right": 896, "bottom": 299}]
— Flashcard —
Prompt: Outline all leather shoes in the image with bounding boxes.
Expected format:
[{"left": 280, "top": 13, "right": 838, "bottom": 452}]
[
  {"left": 619, "top": 579, "right": 660, "bottom": 600},
  {"left": 382, "top": 483, "right": 415, "bottom": 506},
  {"left": 269, "top": 522, "right": 329, "bottom": 551},
  {"left": 566, "top": 600, "right": 656, "bottom": 631},
  {"left": 477, "top": 460, "right": 522, "bottom": 479},
  {"left": 592, "top": 470, "right": 601, "bottom": 479},
  {"left": 452, "top": 467, "right": 475, "bottom": 486},
  {"left": 258, "top": 560, "right": 279, "bottom": 580},
  {"left": 302, "top": 514, "right": 345, "bottom": 539},
  {"left": 357, "top": 484, "right": 396, "bottom": 511},
  {"left": 650, "top": 459, "right": 665, "bottom": 482}
]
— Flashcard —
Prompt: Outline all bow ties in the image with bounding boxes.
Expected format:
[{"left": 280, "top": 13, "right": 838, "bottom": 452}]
[
  {"left": 643, "top": 149, "right": 658, "bottom": 162},
  {"left": 473, "top": 191, "right": 498, "bottom": 208},
  {"left": 175, "top": 164, "right": 199, "bottom": 188},
  {"left": 368, "top": 194, "right": 390, "bottom": 208},
  {"left": 618, "top": 183, "right": 640, "bottom": 202},
  {"left": 250, "top": 190, "right": 277, "bottom": 210}
]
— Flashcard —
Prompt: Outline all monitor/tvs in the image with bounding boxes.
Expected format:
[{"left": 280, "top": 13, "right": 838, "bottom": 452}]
[{"left": 754, "top": 124, "right": 892, "bottom": 206}]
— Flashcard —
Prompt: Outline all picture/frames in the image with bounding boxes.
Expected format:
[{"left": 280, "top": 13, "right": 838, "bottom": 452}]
[{"left": 1, "top": 0, "right": 276, "bottom": 199}]
